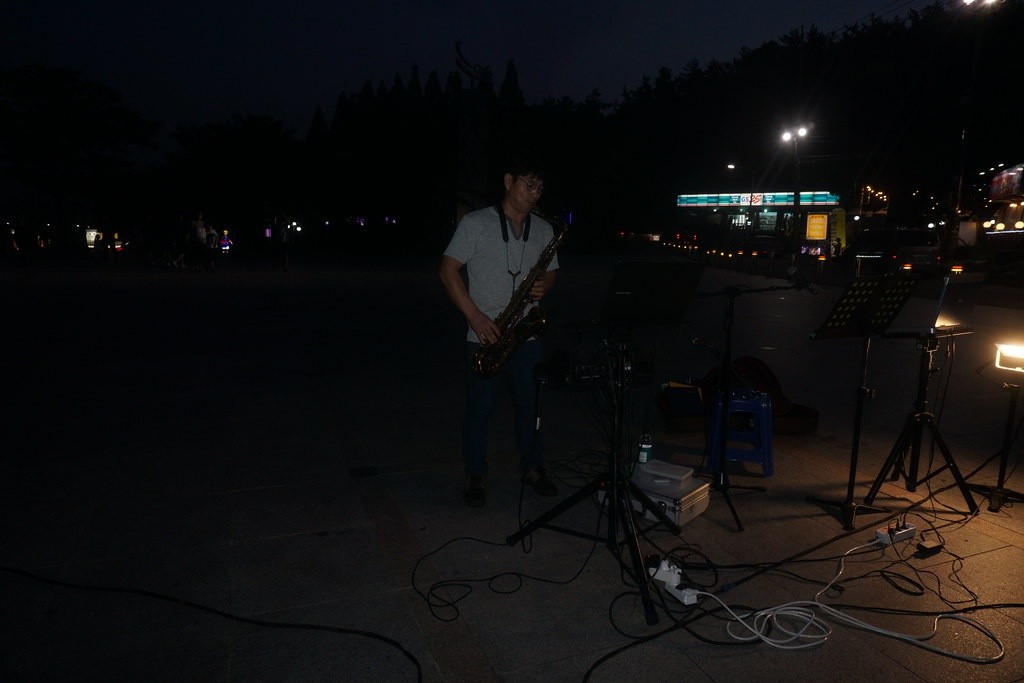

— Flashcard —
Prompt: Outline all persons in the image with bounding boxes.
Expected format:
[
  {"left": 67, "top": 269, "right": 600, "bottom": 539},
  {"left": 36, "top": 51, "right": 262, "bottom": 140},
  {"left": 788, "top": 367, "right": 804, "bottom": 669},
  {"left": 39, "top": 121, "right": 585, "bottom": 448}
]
[
  {"left": 783, "top": 215, "right": 788, "bottom": 230},
  {"left": 438, "top": 157, "right": 560, "bottom": 508},
  {"left": 832, "top": 237, "right": 841, "bottom": 256}
]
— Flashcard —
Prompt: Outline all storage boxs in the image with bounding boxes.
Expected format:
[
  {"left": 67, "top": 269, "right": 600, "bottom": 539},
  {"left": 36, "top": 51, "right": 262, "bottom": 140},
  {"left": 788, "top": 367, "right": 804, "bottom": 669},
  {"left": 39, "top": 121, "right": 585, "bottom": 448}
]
[
  {"left": 661, "top": 376, "right": 708, "bottom": 416},
  {"left": 598, "top": 457, "right": 713, "bottom": 528}
]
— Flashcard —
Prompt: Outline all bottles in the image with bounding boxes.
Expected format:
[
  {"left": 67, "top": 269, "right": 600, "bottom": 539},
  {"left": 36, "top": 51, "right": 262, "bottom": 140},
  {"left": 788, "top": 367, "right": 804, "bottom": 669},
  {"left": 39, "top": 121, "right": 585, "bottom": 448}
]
[{"left": 636, "top": 427, "right": 653, "bottom": 465}]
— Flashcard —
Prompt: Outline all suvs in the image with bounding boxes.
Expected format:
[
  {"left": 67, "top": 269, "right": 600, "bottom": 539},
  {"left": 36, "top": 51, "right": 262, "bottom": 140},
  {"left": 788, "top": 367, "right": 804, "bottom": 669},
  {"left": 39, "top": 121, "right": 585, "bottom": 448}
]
[{"left": 840, "top": 225, "right": 943, "bottom": 278}]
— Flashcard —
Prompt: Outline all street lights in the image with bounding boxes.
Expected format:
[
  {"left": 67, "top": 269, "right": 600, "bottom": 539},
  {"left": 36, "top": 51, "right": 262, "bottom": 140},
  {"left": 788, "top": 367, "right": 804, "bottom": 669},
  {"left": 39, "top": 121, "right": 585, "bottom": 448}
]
[{"left": 780, "top": 125, "right": 808, "bottom": 253}]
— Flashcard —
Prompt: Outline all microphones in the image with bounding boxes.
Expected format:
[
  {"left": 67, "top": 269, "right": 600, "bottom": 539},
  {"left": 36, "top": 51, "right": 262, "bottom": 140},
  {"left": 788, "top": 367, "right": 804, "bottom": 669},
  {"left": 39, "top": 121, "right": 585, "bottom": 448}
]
[{"left": 787, "top": 267, "right": 817, "bottom": 295}]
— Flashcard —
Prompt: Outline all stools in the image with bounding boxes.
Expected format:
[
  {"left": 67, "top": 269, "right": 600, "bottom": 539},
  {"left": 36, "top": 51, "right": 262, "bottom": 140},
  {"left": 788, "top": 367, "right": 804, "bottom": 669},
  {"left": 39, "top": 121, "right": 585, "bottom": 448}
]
[{"left": 707, "top": 388, "right": 775, "bottom": 477}]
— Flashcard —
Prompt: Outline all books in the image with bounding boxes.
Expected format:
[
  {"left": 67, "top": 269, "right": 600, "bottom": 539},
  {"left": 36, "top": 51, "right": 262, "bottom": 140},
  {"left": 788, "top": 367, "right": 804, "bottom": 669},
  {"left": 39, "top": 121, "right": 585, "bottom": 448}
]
[{"left": 645, "top": 460, "right": 693, "bottom": 481}]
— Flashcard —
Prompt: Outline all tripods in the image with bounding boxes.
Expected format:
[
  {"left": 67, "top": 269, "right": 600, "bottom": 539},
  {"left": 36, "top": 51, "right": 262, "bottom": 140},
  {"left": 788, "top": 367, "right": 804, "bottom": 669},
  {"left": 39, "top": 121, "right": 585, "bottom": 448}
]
[
  {"left": 694, "top": 282, "right": 802, "bottom": 538},
  {"left": 506, "top": 328, "right": 685, "bottom": 627},
  {"left": 808, "top": 250, "right": 980, "bottom": 529}
]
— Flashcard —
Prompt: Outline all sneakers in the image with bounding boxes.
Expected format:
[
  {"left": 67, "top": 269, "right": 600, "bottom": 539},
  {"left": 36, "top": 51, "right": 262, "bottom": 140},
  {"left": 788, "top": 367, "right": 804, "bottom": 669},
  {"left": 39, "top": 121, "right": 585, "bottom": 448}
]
[
  {"left": 464, "top": 473, "right": 487, "bottom": 507},
  {"left": 523, "top": 472, "right": 561, "bottom": 501}
]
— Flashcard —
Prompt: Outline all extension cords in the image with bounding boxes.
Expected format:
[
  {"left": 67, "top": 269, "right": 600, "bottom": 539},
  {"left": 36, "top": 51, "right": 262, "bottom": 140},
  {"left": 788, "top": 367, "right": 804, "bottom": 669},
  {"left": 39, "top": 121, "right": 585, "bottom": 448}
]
[
  {"left": 663, "top": 580, "right": 697, "bottom": 605},
  {"left": 876, "top": 522, "right": 915, "bottom": 544}
]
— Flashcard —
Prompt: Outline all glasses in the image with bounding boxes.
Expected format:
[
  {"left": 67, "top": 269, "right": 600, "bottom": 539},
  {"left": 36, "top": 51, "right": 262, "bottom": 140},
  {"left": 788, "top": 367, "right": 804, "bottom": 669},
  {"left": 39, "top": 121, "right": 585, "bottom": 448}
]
[{"left": 510, "top": 173, "right": 545, "bottom": 194}]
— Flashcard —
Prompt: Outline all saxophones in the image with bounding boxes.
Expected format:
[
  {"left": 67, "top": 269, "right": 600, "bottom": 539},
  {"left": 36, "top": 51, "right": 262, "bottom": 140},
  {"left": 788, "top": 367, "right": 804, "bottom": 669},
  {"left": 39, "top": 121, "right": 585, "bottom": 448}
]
[{"left": 470, "top": 203, "right": 572, "bottom": 372}]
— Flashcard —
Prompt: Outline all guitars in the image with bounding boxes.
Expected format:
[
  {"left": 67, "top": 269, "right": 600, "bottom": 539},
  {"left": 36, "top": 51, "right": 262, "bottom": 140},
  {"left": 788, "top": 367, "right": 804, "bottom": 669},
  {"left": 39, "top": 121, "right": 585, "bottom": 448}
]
[{"left": 687, "top": 332, "right": 772, "bottom": 413}]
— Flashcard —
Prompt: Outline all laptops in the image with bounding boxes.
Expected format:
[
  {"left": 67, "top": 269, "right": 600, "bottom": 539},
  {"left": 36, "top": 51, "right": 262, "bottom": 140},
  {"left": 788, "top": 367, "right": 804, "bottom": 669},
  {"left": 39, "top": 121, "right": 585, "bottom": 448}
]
[
  {"left": 564, "top": 261, "right": 706, "bottom": 327},
  {"left": 887, "top": 273, "right": 987, "bottom": 334}
]
